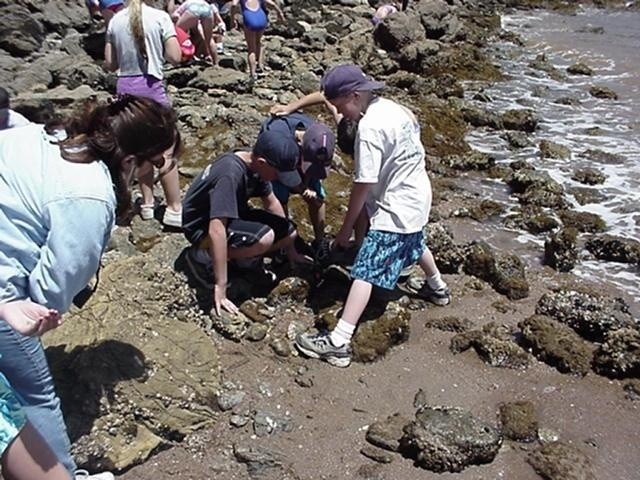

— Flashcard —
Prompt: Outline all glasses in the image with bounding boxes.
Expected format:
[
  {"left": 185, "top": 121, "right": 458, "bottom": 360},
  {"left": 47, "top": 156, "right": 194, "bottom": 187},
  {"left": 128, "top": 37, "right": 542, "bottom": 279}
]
[{"left": 136, "top": 153, "right": 165, "bottom": 169}]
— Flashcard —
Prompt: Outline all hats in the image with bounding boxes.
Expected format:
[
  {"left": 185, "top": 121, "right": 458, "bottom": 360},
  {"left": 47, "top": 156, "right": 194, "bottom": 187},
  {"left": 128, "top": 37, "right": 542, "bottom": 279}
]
[
  {"left": 319, "top": 63, "right": 387, "bottom": 100},
  {"left": 298, "top": 122, "right": 336, "bottom": 180},
  {"left": 253, "top": 129, "right": 303, "bottom": 187}
]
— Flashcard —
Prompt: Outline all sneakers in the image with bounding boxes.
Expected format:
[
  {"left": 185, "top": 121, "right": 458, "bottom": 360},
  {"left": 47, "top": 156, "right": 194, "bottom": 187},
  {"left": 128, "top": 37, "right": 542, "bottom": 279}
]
[
  {"left": 291, "top": 231, "right": 332, "bottom": 269},
  {"left": 162, "top": 202, "right": 186, "bottom": 228},
  {"left": 75, "top": 468, "right": 115, "bottom": 480},
  {"left": 186, "top": 248, "right": 232, "bottom": 290},
  {"left": 137, "top": 193, "right": 156, "bottom": 221},
  {"left": 295, "top": 332, "right": 352, "bottom": 368},
  {"left": 406, "top": 276, "right": 453, "bottom": 308}
]
